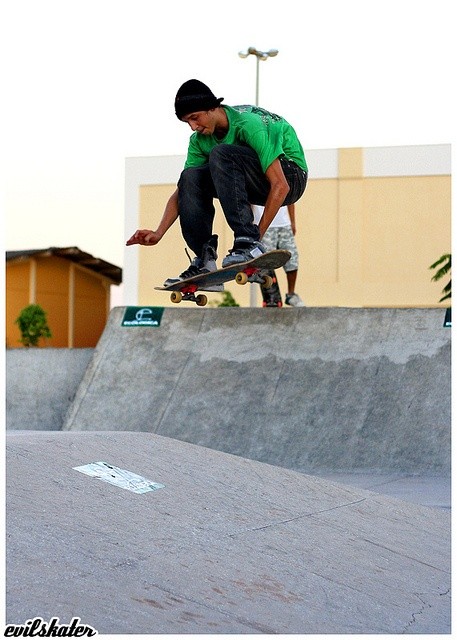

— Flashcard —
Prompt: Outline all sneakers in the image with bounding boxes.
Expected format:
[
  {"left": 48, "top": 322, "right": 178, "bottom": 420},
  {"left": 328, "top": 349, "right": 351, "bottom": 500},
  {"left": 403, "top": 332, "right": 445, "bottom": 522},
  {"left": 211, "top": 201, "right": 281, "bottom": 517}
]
[
  {"left": 222, "top": 237, "right": 267, "bottom": 268},
  {"left": 164, "top": 248, "right": 225, "bottom": 292},
  {"left": 284, "top": 293, "right": 306, "bottom": 307}
]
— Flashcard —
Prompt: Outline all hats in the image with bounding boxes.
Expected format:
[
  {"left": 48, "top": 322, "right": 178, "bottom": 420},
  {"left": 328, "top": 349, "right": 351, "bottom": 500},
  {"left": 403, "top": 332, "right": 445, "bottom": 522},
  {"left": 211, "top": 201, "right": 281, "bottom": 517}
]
[{"left": 174, "top": 78, "right": 225, "bottom": 121}]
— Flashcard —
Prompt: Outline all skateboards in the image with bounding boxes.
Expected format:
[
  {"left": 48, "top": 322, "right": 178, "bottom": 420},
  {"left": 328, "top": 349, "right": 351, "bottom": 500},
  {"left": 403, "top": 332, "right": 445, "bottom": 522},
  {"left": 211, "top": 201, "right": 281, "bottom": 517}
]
[{"left": 153, "top": 249, "right": 291, "bottom": 307}]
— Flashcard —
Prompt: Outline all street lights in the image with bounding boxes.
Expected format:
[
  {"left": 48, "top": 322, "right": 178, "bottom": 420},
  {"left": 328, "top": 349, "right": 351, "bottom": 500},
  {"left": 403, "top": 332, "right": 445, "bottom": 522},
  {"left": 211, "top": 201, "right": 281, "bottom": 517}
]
[{"left": 237, "top": 46, "right": 278, "bottom": 107}]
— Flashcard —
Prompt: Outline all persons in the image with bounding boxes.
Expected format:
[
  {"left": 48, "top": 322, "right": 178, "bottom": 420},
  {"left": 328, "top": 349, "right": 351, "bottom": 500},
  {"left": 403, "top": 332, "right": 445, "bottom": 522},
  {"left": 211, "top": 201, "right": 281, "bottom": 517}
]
[
  {"left": 124, "top": 79, "right": 308, "bottom": 289},
  {"left": 248, "top": 200, "right": 306, "bottom": 308}
]
